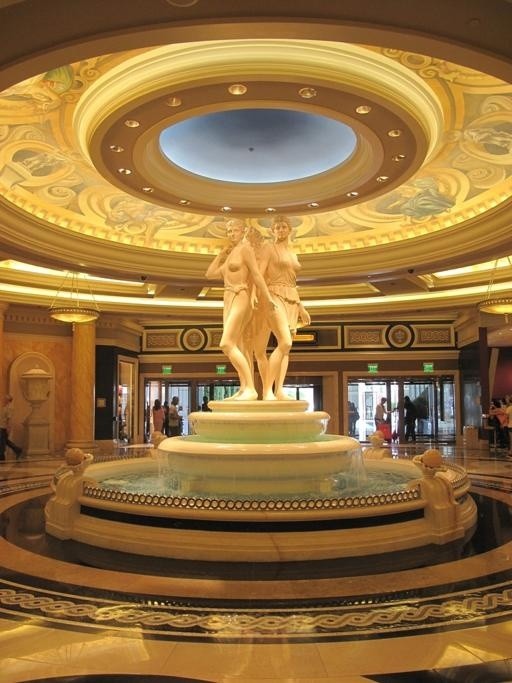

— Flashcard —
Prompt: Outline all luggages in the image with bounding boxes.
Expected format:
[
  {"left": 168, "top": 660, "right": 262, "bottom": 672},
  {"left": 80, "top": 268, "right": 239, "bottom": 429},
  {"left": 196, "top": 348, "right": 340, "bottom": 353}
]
[{"left": 376, "top": 423, "right": 392, "bottom": 442}]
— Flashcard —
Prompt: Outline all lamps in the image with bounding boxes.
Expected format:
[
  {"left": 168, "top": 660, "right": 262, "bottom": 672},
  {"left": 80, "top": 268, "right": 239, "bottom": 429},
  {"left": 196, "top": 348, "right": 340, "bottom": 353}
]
[
  {"left": 473, "top": 239, "right": 511, "bottom": 326},
  {"left": 47, "top": 267, "right": 102, "bottom": 330}
]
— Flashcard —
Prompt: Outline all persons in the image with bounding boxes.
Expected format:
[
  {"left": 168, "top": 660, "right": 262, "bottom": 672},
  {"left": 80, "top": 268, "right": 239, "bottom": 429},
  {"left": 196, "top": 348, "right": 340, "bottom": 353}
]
[
  {"left": 374, "top": 397, "right": 394, "bottom": 432},
  {"left": 204, "top": 214, "right": 312, "bottom": 401},
  {"left": 0, "top": 394, "right": 22, "bottom": 461},
  {"left": 348, "top": 401, "right": 359, "bottom": 435},
  {"left": 202, "top": 395, "right": 211, "bottom": 412},
  {"left": 147, "top": 395, "right": 183, "bottom": 438},
  {"left": 403, "top": 396, "right": 418, "bottom": 444},
  {"left": 488, "top": 393, "right": 512, "bottom": 457}
]
